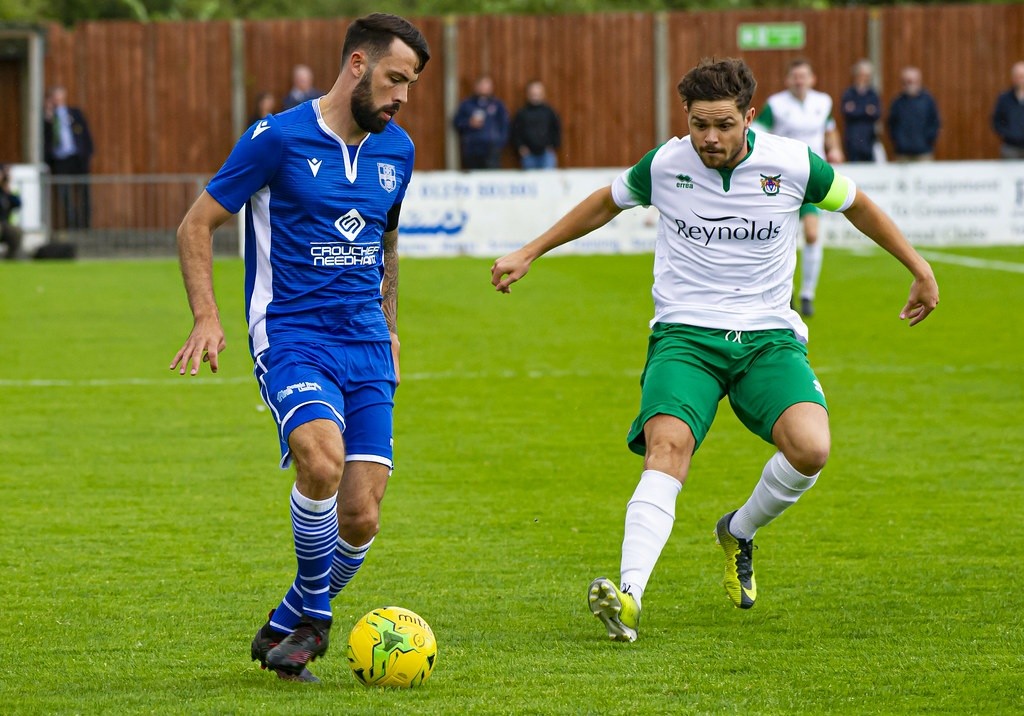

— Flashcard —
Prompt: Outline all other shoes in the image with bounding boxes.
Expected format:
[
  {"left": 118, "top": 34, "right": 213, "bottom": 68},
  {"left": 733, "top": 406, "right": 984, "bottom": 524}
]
[{"left": 799, "top": 297, "right": 814, "bottom": 314}]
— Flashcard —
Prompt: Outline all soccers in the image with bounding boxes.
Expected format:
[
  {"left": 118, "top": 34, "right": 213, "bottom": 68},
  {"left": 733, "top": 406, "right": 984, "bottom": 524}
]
[{"left": 347, "top": 607, "right": 437, "bottom": 688}]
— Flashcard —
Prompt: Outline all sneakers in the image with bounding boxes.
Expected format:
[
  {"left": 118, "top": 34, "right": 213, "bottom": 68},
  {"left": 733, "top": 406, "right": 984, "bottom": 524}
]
[
  {"left": 713, "top": 509, "right": 757, "bottom": 610},
  {"left": 251, "top": 610, "right": 295, "bottom": 670},
  {"left": 266, "top": 614, "right": 331, "bottom": 676},
  {"left": 587, "top": 576, "right": 640, "bottom": 643}
]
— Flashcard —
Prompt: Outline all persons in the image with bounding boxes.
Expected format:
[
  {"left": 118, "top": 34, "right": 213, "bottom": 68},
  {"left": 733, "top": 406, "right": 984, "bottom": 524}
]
[
  {"left": 510, "top": 78, "right": 561, "bottom": 170},
  {"left": 42, "top": 83, "right": 93, "bottom": 231},
  {"left": 750, "top": 61, "right": 844, "bottom": 319},
  {"left": 992, "top": 60, "right": 1024, "bottom": 159},
  {"left": 488, "top": 59, "right": 938, "bottom": 644},
  {"left": 246, "top": 92, "right": 277, "bottom": 130},
  {"left": 280, "top": 63, "right": 323, "bottom": 113},
  {"left": 886, "top": 67, "right": 941, "bottom": 162},
  {"left": 452, "top": 73, "right": 512, "bottom": 169},
  {"left": 0, "top": 168, "right": 21, "bottom": 259},
  {"left": 171, "top": 14, "right": 430, "bottom": 682},
  {"left": 841, "top": 61, "right": 881, "bottom": 161}
]
